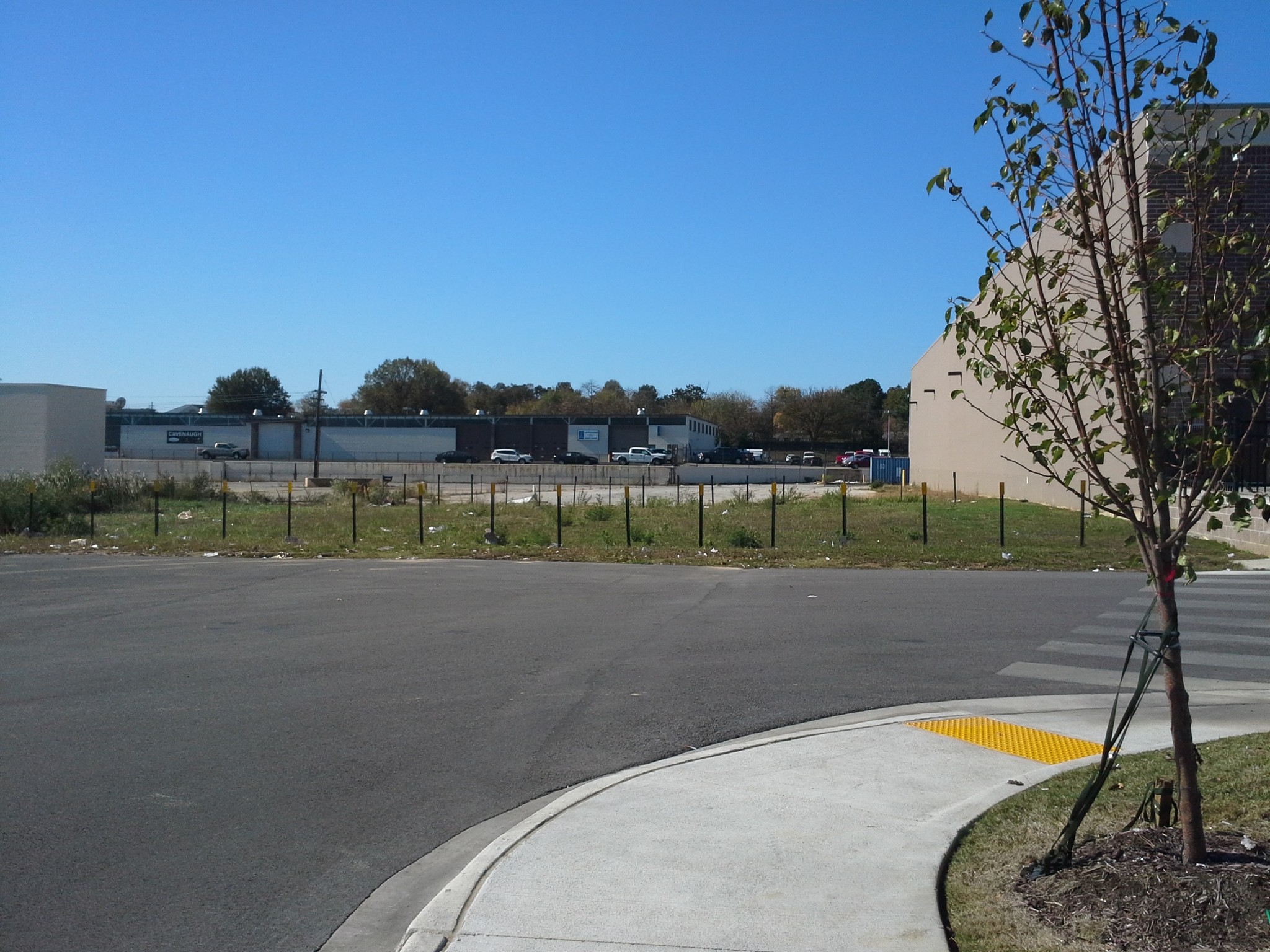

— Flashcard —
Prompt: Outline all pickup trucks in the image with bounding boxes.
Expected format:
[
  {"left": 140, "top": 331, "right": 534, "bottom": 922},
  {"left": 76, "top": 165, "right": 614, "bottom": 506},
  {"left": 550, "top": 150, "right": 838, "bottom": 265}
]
[
  {"left": 197, "top": 443, "right": 250, "bottom": 459},
  {"left": 699, "top": 447, "right": 749, "bottom": 464},
  {"left": 612, "top": 447, "right": 666, "bottom": 466},
  {"left": 836, "top": 451, "right": 878, "bottom": 467}
]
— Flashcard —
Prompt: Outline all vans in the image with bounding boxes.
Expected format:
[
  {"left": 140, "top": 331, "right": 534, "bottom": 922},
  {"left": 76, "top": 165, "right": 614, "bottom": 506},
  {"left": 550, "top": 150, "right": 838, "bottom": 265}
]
[
  {"left": 862, "top": 449, "right": 891, "bottom": 457},
  {"left": 803, "top": 451, "right": 815, "bottom": 463}
]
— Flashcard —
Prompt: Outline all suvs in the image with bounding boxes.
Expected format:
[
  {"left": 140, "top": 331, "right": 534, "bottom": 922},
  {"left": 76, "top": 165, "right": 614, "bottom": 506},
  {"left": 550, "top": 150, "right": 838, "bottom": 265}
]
[
  {"left": 646, "top": 449, "right": 677, "bottom": 464},
  {"left": 789, "top": 456, "right": 801, "bottom": 466},
  {"left": 810, "top": 457, "right": 822, "bottom": 466},
  {"left": 491, "top": 449, "right": 533, "bottom": 464},
  {"left": 785, "top": 454, "right": 795, "bottom": 462}
]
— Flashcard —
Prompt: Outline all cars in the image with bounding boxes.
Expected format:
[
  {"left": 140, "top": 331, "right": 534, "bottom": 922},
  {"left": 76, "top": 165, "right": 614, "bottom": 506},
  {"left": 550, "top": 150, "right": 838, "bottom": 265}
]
[
  {"left": 843, "top": 454, "right": 870, "bottom": 464},
  {"left": 553, "top": 452, "right": 598, "bottom": 465},
  {"left": 847, "top": 456, "right": 870, "bottom": 469},
  {"left": 435, "top": 451, "right": 481, "bottom": 464}
]
